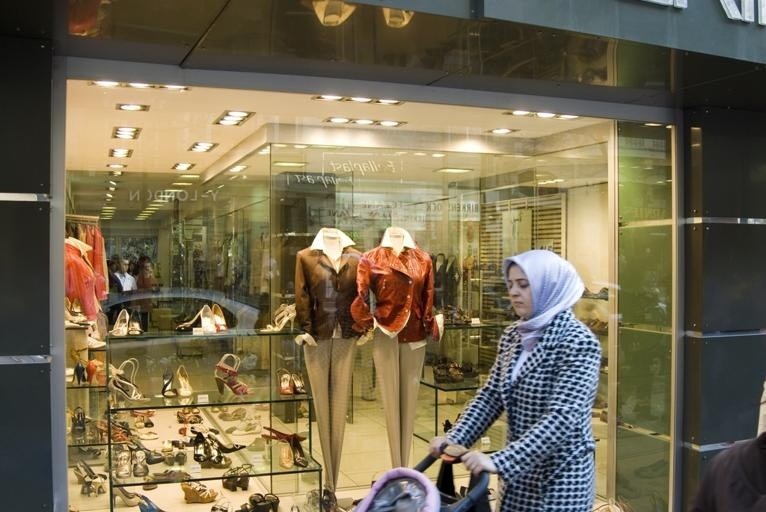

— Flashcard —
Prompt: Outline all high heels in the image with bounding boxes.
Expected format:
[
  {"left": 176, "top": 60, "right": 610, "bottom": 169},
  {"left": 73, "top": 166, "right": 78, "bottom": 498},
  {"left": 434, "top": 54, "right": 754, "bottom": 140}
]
[
  {"left": 63, "top": 297, "right": 110, "bottom": 497},
  {"left": 110, "top": 354, "right": 306, "bottom": 512},
  {"left": 260, "top": 304, "right": 296, "bottom": 333}
]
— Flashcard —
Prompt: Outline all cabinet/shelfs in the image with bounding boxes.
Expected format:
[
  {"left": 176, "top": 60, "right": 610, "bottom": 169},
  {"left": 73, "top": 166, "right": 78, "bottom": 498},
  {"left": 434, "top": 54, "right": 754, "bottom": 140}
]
[
  {"left": 59, "top": 316, "right": 110, "bottom": 512},
  {"left": 101, "top": 323, "right": 326, "bottom": 512},
  {"left": 406, "top": 314, "right": 505, "bottom": 506}
]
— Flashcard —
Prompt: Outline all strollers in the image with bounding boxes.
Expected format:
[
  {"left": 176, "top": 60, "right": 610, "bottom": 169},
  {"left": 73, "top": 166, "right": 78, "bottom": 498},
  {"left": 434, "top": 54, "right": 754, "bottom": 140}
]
[{"left": 352, "top": 437, "right": 494, "bottom": 512}]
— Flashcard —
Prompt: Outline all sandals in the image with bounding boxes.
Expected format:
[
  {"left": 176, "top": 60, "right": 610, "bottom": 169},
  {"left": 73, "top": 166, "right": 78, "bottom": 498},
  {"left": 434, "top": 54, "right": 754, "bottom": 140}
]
[
  {"left": 435, "top": 305, "right": 473, "bottom": 325},
  {"left": 433, "top": 357, "right": 479, "bottom": 383}
]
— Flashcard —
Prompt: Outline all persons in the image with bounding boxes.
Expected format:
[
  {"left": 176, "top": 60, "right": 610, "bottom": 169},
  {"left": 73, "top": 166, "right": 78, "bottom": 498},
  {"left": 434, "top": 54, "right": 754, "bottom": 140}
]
[
  {"left": 106, "top": 255, "right": 158, "bottom": 331},
  {"left": 430, "top": 250, "right": 602, "bottom": 511},
  {"left": 295, "top": 227, "right": 373, "bottom": 491},
  {"left": 351, "top": 227, "right": 444, "bottom": 467},
  {"left": 694, "top": 431, "right": 766, "bottom": 512}
]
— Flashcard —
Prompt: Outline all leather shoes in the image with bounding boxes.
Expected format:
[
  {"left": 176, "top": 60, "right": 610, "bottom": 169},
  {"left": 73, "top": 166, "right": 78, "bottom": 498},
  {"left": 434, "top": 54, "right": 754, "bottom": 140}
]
[
  {"left": 176, "top": 304, "right": 227, "bottom": 335},
  {"left": 129, "top": 310, "right": 144, "bottom": 336},
  {"left": 112, "top": 309, "right": 129, "bottom": 337}
]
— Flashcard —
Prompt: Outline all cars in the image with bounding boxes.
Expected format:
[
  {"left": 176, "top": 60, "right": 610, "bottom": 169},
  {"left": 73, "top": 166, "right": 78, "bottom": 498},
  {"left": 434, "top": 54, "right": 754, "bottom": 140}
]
[
  {"left": 582, "top": 282, "right": 668, "bottom": 382},
  {"left": 94, "top": 286, "right": 269, "bottom": 384}
]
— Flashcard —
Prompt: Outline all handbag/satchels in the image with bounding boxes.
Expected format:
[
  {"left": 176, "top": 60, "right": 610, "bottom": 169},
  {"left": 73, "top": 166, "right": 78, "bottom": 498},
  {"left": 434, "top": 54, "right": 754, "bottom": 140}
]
[{"left": 437, "top": 472, "right": 493, "bottom": 511}]
